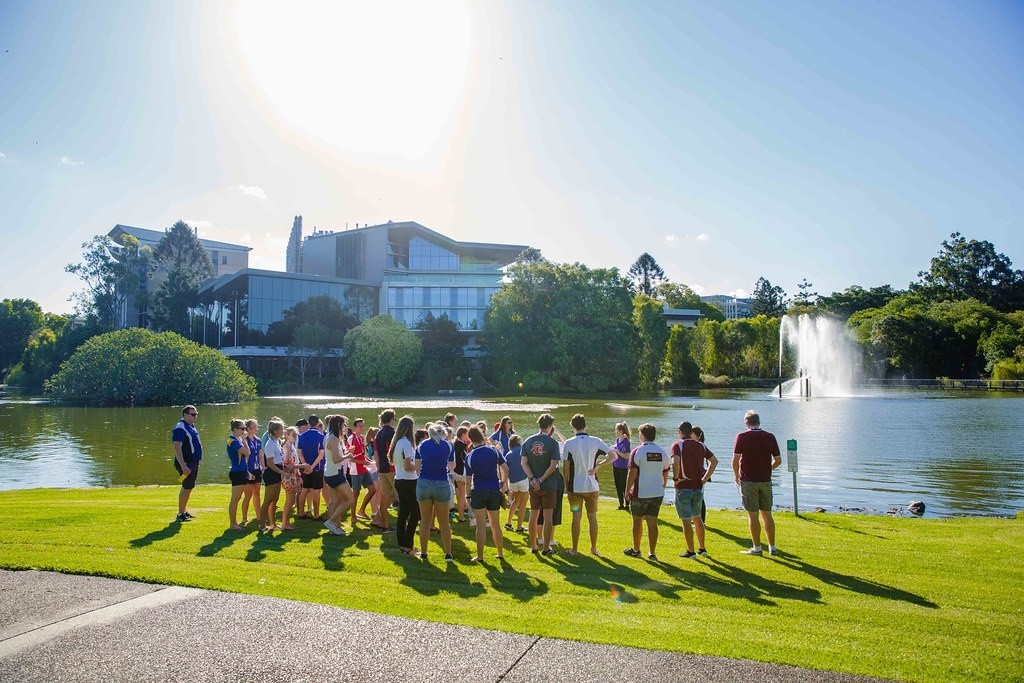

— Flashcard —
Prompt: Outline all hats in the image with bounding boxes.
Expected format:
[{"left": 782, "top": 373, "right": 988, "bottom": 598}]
[{"left": 296, "top": 418, "right": 309, "bottom": 428}]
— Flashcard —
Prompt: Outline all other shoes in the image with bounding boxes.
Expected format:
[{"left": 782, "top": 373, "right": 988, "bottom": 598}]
[
  {"left": 430, "top": 528, "right": 441, "bottom": 534},
  {"left": 591, "top": 550, "right": 599, "bottom": 556},
  {"left": 549, "top": 540, "right": 557, "bottom": 545},
  {"left": 566, "top": 549, "right": 576, "bottom": 555},
  {"left": 471, "top": 556, "right": 483, "bottom": 562},
  {"left": 230, "top": 524, "right": 242, "bottom": 530},
  {"left": 494, "top": 554, "right": 503, "bottom": 558},
  {"left": 769, "top": 549, "right": 779, "bottom": 556},
  {"left": 504, "top": 523, "right": 514, "bottom": 532},
  {"left": 538, "top": 538, "right": 544, "bottom": 545},
  {"left": 458, "top": 518, "right": 468, "bottom": 522},
  {"left": 515, "top": 525, "right": 528, "bottom": 532},
  {"left": 469, "top": 518, "right": 477, "bottom": 526},
  {"left": 444, "top": 553, "right": 453, "bottom": 561},
  {"left": 282, "top": 524, "right": 296, "bottom": 530},
  {"left": 370, "top": 522, "right": 396, "bottom": 531},
  {"left": 740, "top": 548, "right": 762, "bottom": 556},
  {"left": 313, "top": 515, "right": 326, "bottom": 521},
  {"left": 419, "top": 553, "right": 428, "bottom": 560},
  {"left": 297, "top": 515, "right": 311, "bottom": 520}
]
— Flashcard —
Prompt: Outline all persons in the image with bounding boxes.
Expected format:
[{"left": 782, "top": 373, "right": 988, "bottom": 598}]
[
  {"left": 670, "top": 421, "right": 719, "bottom": 559},
  {"left": 689, "top": 426, "right": 712, "bottom": 524},
  {"left": 561, "top": 413, "right": 618, "bottom": 556},
  {"left": 171, "top": 405, "right": 204, "bottom": 523},
  {"left": 731, "top": 410, "right": 782, "bottom": 556},
  {"left": 623, "top": 423, "right": 671, "bottom": 560},
  {"left": 225, "top": 407, "right": 566, "bottom": 566},
  {"left": 610, "top": 421, "right": 632, "bottom": 510}
]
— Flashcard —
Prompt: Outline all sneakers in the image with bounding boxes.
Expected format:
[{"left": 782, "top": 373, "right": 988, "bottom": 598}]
[
  {"left": 624, "top": 548, "right": 641, "bottom": 557},
  {"left": 697, "top": 548, "right": 706, "bottom": 555},
  {"left": 542, "top": 549, "right": 556, "bottom": 555},
  {"left": 648, "top": 552, "right": 657, "bottom": 560},
  {"left": 177, "top": 511, "right": 196, "bottom": 522},
  {"left": 258, "top": 525, "right": 283, "bottom": 531},
  {"left": 678, "top": 551, "right": 696, "bottom": 558},
  {"left": 324, "top": 520, "right": 348, "bottom": 535},
  {"left": 532, "top": 546, "right": 539, "bottom": 554}
]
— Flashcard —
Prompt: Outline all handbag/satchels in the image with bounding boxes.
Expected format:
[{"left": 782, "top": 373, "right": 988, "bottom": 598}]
[{"left": 499, "top": 491, "right": 507, "bottom": 510}]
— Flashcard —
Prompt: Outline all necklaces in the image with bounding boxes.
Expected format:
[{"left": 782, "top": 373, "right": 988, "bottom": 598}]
[{"left": 751, "top": 428, "right": 761, "bottom": 430}]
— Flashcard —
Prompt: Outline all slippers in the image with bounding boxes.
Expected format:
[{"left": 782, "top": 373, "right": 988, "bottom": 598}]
[{"left": 356, "top": 514, "right": 371, "bottom": 520}]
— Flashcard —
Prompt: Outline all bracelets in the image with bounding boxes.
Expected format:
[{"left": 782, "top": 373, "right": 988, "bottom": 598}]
[
  {"left": 465, "top": 496, "right": 471, "bottom": 499},
  {"left": 536, "top": 478, "right": 542, "bottom": 483},
  {"left": 530, "top": 476, "right": 535, "bottom": 481},
  {"left": 673, "top": 477, "right": 679, "bottom": 481}
]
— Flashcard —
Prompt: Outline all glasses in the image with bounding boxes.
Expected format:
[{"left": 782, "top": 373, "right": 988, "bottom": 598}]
[
  {"left": 187, "top": 411, "right": 200, "bottom": 417},
  {"left": 288, "top": 434, "right": 300, "bottom": 437},
  {"left": 506, "top": 422, "right": 513, "bottom": 424},
  {"left": 236, "top": 427, "right": 247, "bottom": 431}
]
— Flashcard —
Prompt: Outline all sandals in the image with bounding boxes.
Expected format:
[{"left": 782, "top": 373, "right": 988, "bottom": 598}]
[{"left": 400, "top": 546, "right": 418, "bottom": 555}]
